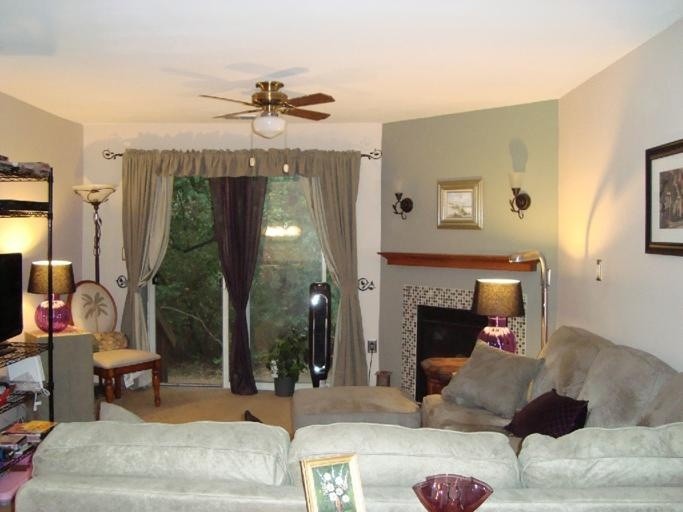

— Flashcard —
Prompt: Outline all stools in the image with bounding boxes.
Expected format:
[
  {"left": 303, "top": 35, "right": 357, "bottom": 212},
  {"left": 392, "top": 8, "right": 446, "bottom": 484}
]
[{"left": 292, "top": 386, "right": 422, "bottom": 437}]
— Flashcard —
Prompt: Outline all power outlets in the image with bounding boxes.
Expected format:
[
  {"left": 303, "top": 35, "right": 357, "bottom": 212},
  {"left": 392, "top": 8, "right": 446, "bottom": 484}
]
[{"left": 368, "top": 341, "right": 377, "bottom": 353}]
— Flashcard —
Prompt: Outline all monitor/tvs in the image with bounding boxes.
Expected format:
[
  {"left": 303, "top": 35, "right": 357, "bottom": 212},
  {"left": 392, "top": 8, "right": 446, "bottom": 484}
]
[{"left": 0, "top": 252, "right": 23, "bottom": 357}]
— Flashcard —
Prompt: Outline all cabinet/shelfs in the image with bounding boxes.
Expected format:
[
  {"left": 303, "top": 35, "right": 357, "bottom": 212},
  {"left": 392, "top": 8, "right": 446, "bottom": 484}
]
[{"left": 0, "top": 168, "right": 54, "bottom": 473}]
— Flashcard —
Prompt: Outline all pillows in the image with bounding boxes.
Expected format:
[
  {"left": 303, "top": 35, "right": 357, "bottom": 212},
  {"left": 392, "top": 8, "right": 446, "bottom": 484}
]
[
  {"left": 99, "top": 400, "right": 142, "bottom": 421},
  {"left": 94, "top": 331, "right": 128, "bottom": 351},
  {"left": 32, "top": 421, "right": 291, "bottom": 487},
  {"left": 519, "top": 422, "right": 682, "bottom": 489},
  {"left": 441, "top": 324, "right": 683, "bottom": 439},
  {"left": 290, "top": 422, "right": 519, "bottom": 489}
]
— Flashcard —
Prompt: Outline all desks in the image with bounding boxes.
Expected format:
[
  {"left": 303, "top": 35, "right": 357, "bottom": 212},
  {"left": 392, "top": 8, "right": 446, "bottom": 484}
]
[
  {"left": 421, "top": 357, "right": 469, "bottom": 395},
  {"left": 25, "top": 328, "right": 96, "bottom": 423}
]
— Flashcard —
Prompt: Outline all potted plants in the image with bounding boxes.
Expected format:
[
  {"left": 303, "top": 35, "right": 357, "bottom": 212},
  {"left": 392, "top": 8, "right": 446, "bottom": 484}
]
[{"left": 266, "top": 327, "right": 311, "bottom": 398}]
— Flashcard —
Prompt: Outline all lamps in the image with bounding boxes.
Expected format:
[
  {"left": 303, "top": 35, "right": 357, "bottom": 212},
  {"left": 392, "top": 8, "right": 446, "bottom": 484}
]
[
  {"left": 472, "top": 278, "right": 524, "bottom": 353},
  {"left": 509, "top": 250, "right": 548, "bottom": 349},
  {"left": 27, "top": 261, "right": 75, "bottom": 333},
  {"left": 509, "top": 173, "right": 531, "bottom": 220},
  {"left": 251, "top": 105, "right": 287, "bottom": 139},
  {"left": 72, "top": 184, "right": 117, "bottom": 395},
  {"left": 392, "top": 192, "right": 413, "bottom": 220}
]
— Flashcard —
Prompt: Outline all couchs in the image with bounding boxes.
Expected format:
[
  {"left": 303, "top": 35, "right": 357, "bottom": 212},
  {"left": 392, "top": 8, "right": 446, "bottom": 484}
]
[{"left": 13, "top": 394, "right": 683, "bottom": 512}]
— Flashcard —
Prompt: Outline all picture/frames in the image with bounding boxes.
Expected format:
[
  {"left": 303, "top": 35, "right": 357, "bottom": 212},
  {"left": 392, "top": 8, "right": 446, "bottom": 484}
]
[
  {"left": 645, "top": 138, "right": 683, "bottom": 256},
  {"left": 300, "top": 453, "right": 366, "bottom": 512},
  {"left": 436, "top": 177, "right": 484, "bottom": 229}
]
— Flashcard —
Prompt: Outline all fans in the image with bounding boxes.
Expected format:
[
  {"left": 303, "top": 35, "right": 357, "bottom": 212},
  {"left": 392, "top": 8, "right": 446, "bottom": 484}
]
[{"left": 200, "top": 81, "right": 335, "bottom": 121}]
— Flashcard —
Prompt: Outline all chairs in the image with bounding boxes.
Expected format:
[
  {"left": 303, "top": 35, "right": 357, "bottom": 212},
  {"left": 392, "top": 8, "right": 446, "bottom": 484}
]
[{"left": 67, "top": 281, "right": 161, "bottom": 407}]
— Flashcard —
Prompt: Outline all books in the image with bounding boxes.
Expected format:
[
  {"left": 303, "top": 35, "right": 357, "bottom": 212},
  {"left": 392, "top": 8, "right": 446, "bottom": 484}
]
[{"left": 0, "top": 420, "right": 58, "bottom": 462}]
